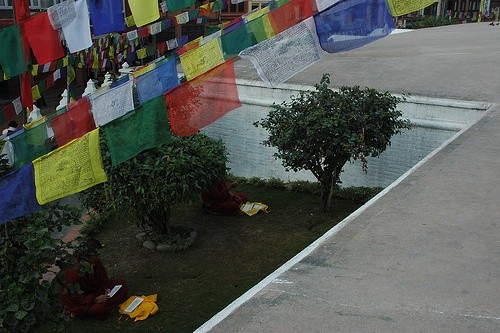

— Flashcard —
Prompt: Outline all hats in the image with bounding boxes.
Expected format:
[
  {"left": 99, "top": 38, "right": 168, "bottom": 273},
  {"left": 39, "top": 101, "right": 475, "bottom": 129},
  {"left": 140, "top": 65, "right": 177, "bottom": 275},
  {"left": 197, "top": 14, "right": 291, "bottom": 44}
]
[{"left": 7, "top": 120, "right": 18, "bottom": 127}]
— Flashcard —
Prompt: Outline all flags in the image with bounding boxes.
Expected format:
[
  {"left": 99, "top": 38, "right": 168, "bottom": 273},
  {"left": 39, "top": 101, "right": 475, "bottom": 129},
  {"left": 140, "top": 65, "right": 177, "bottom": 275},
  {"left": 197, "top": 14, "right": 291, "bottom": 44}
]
[
  {"left": 0, "top": 0, "right": 335, "bottom": 172},
  {"left": 386, "top": 0, "right": 438, "bottom": 16},
  {"left": 32, "top": 127, "right": 110, "bottom": 206},
  {"left": 0, "top": 161, "right": 40, "bottom": 226},
  {"left": 315, "top": 0, "right": 395, "bottom": 54}
]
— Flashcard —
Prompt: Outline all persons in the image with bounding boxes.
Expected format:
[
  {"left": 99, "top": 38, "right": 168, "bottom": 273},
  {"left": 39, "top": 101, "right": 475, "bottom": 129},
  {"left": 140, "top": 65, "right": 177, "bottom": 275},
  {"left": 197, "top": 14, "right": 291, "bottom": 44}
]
[
  {"left": 62, "top": 238, "right": 127, "bottom": 320},
  {"left": 201, "top": 179, "right": 246, "bottom": 213}
]
